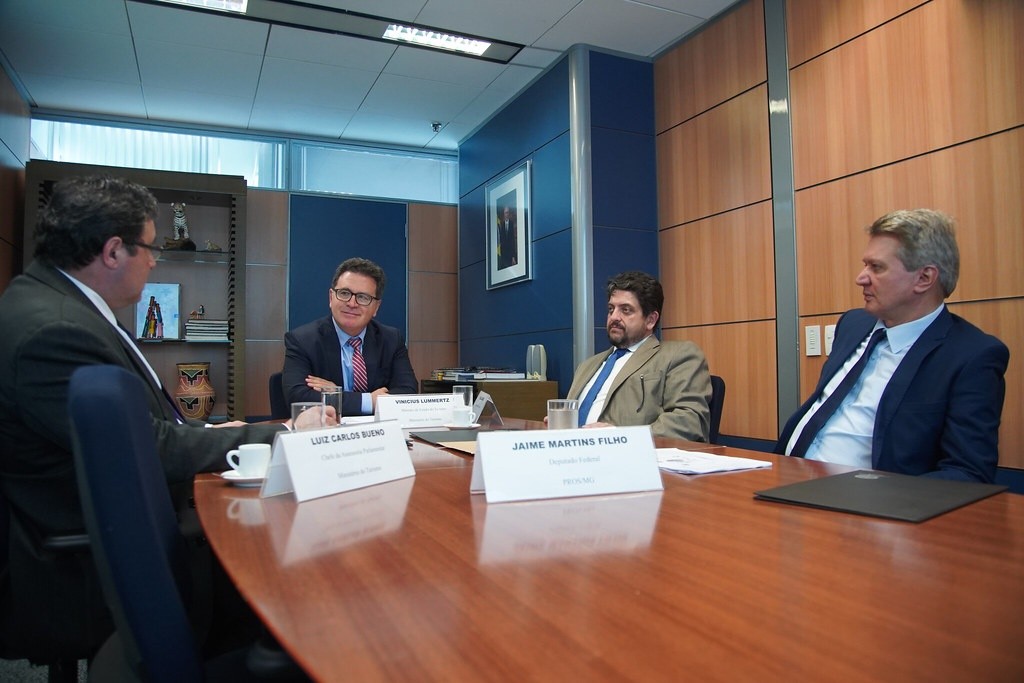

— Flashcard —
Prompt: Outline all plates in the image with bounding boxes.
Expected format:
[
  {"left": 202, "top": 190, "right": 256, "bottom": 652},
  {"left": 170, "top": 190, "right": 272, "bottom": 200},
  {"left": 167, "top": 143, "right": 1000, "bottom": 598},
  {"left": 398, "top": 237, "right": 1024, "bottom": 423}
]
[
  {"left": 443, "top": 423, "right": 482, "bottom": 430},
  {"left": 221, "top": 470, "right": 264, "bottom": 486},
  {"left": 340, "top": 416, "right": 375, "bottom": 424}
]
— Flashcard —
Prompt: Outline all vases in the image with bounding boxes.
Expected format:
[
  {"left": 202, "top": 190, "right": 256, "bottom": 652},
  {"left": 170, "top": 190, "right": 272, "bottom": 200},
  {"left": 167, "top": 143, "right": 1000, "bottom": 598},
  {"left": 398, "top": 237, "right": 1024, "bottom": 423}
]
[{"left": 174, "top": 361, "right": 217, "bottom": 422}]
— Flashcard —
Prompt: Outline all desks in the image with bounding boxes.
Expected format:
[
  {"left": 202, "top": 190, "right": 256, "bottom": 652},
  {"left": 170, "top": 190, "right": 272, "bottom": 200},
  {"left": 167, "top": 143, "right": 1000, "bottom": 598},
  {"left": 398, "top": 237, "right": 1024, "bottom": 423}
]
[
  {"left": 192, "top": 416, "right": 1024, "bottom": 683},
  {"left": 421, "top": 379, "right": 558, "bottom": 422}
]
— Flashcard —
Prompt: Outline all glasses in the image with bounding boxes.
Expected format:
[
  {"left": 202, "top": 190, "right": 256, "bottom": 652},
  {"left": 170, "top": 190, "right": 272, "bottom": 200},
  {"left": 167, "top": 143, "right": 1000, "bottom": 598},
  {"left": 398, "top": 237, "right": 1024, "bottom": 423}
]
[
  {"left": 123, "top": 236, "right": 165, "bottom": 263},
  {"left": 331, "top": 286, "right": 380, "bottom": 305}
]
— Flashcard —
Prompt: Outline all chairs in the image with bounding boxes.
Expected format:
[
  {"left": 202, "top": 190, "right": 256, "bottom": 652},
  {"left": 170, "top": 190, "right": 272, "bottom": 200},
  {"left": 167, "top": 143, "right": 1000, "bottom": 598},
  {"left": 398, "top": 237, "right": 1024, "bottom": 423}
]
[
  {"left": 708, "top": 375, "right": 726, "bottom": 444},
  {"left": 0, "top": 498, "right": 257, "bottom": 683},
  {"left": 268, "top": 372, "right": 291, "bottom": 420},
  {"left": 66, "top": 364, "right": 314, "bottom": 683}
]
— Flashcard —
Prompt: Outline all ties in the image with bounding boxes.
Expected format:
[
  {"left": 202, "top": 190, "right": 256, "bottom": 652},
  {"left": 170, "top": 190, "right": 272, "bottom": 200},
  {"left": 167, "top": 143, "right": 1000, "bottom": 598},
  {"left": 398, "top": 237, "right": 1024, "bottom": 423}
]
[
  {"left": 115, "top": 317, "right": 186, "bottom": 425},
  {"left": 578, "top": 347, "right": 630, "bottom": 427},
  {"left": 790, "top": 328, "right": 888, "bottom": 458},
  {"left": 346, "top": 339, "right": 368, "bottom": 393}
]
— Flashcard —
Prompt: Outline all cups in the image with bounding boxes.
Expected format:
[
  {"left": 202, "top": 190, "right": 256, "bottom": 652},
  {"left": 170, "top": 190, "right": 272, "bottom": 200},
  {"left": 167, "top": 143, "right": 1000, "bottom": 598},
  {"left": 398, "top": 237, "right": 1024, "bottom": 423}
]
[
  {"left": 292, "top": 402, "right": 324, "bottom": 430},
  {"left": 321, "top": 387, "right": 343, "bottom": 426},
  {"left": 226, "top": 443, "right": 271, "bottom": 476},
  {"left": 547, "top": 399, "right": 580, "bottom": 430},
  {"left": 449, "top": 408, "right": 476, "bottom": 425},
  {"left": 452, "top": 386, "right": 472, "bottom": 411}
]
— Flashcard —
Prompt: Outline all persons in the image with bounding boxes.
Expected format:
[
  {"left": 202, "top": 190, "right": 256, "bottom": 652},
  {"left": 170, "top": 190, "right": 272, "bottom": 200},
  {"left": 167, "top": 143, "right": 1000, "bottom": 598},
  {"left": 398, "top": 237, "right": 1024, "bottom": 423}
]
[
  {"left": 282, "top": 257, "right": 420, "bottom": 418},
  {"left": 544, "top": 271, "right": 713, "bottom": 445},
  {"left": 0, "top": 175, "right": 339, "bottom": 683},
  {"left": 773, "top": 208, "right": 1011, "bottom": 486},
  {"left": 500, "top": 206, "right": 516, "bottom": 269}
]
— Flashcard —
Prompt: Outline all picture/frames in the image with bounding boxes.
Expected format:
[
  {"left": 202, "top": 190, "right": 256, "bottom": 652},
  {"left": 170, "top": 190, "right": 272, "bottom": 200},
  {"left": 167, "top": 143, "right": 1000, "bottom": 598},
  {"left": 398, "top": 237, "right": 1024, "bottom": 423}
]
[{"left": 485, "top": 160, "right": 533, "bottom": 292}]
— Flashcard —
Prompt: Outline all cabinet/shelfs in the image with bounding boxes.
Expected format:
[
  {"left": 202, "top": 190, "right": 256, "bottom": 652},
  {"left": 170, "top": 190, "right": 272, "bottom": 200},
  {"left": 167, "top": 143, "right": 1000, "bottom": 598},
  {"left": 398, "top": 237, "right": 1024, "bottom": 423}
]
[{"left": 20, "top": 158, "right": 248, "bottom": 423}]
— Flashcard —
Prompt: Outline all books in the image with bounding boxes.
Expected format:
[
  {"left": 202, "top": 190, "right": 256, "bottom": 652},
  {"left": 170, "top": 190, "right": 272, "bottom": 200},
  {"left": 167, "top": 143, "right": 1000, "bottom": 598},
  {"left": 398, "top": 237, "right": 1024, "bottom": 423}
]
[
  {"left": 429, "top": 367, "right": 465, "bottom": 381},
  {"left": 185, "top": 319, "right": 229, "bottom": 340}
]
